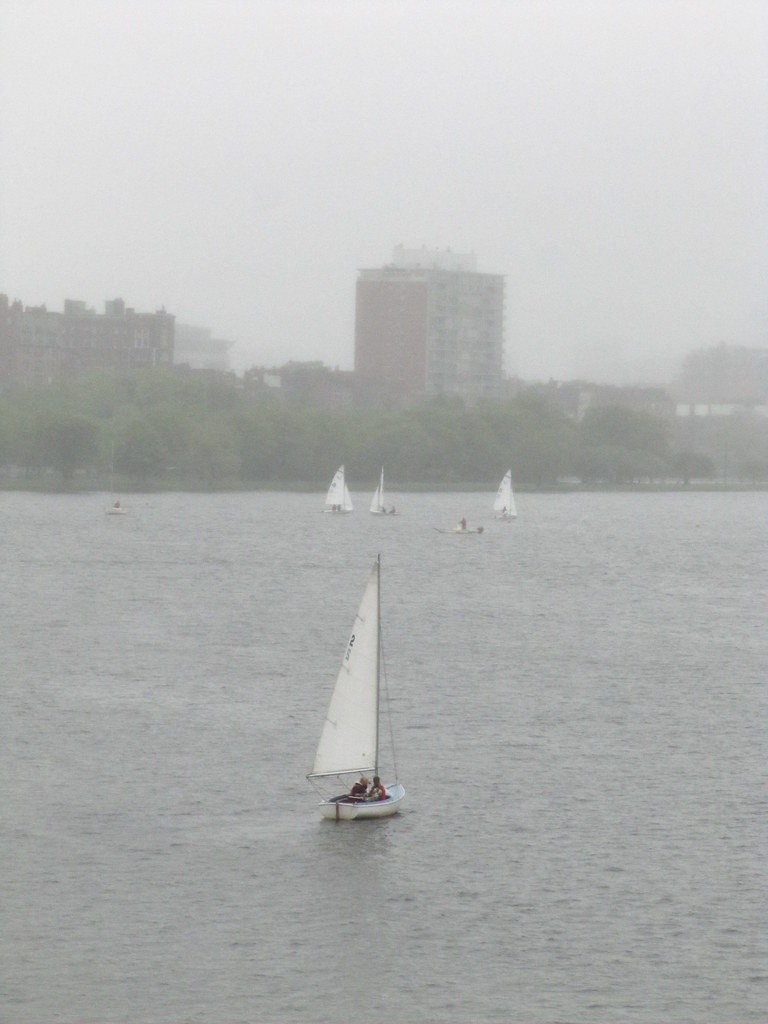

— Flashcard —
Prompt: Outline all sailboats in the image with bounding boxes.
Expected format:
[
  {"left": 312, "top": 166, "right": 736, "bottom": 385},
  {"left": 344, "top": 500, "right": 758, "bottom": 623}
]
[
  {"left": 493, "top": 469, "right": 517, "bottom": 521},
  {"left": 322, "top": 464, "right": 354, "bottom": 513},
  {"left": 368, "top": 465, "right": 395, "bottom": 515},
  {"left": 303, "top": 554, "right": 408, "bottom": 825}
]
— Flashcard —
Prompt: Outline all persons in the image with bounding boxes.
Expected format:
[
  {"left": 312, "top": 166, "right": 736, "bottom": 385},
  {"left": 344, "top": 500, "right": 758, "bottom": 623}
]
[
  {"left": 503, "top": 506, "right": 507, "bottom": 513},
  {"left": 332, "top": 505, "right": 336, "bottom": 510},
  {"left": 350, "top": 777, "right": 368, "bottom": 804},
  {"left": 460, "top": 518, "right": 466, "bottom": 529},
  {"left": 337, "top": 505, "right": 341, "bottom": 510},
  {"left": 113, "top": 500, "right": 120, "bottom": 508},
  {"left": 370, "top": 777, "right": 386, "bottom": 801}
]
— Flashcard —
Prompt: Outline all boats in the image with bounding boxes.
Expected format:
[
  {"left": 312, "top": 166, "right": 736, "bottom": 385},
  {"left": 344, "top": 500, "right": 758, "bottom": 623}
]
[{"left": 434, "top": 522, "right": 484, "bottom": 535}]
